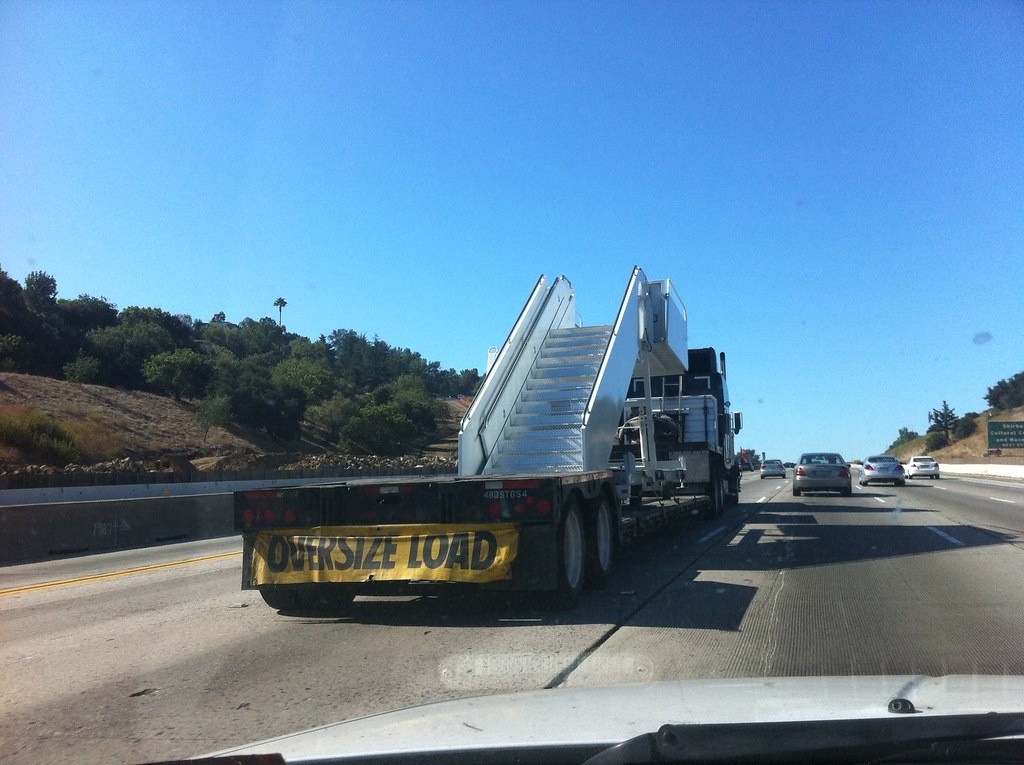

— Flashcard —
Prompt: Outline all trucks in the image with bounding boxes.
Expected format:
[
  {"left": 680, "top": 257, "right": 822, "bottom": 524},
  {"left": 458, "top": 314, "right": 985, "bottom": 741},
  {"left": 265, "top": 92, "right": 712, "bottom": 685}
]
[{"left": 233, "top": 262, "right": 744, "bottom": 613}]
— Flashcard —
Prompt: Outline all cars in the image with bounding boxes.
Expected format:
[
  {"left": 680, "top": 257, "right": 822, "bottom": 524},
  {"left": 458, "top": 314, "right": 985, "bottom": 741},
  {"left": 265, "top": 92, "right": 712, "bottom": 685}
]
[
  {"left": 743, "top": 462, "right": 755, "bottom": 472},
  {"left": 788, "top": 452, "right": 852, "bottom": 497},
  {"left": 760, "top": 459, "right": 786, "bottom": 479},
  {"left": 857, "top": 454, "right": 906, "bottom": 487},
  {"left": 905, "top": 455, "right": 940, "bottom": 480}
]
